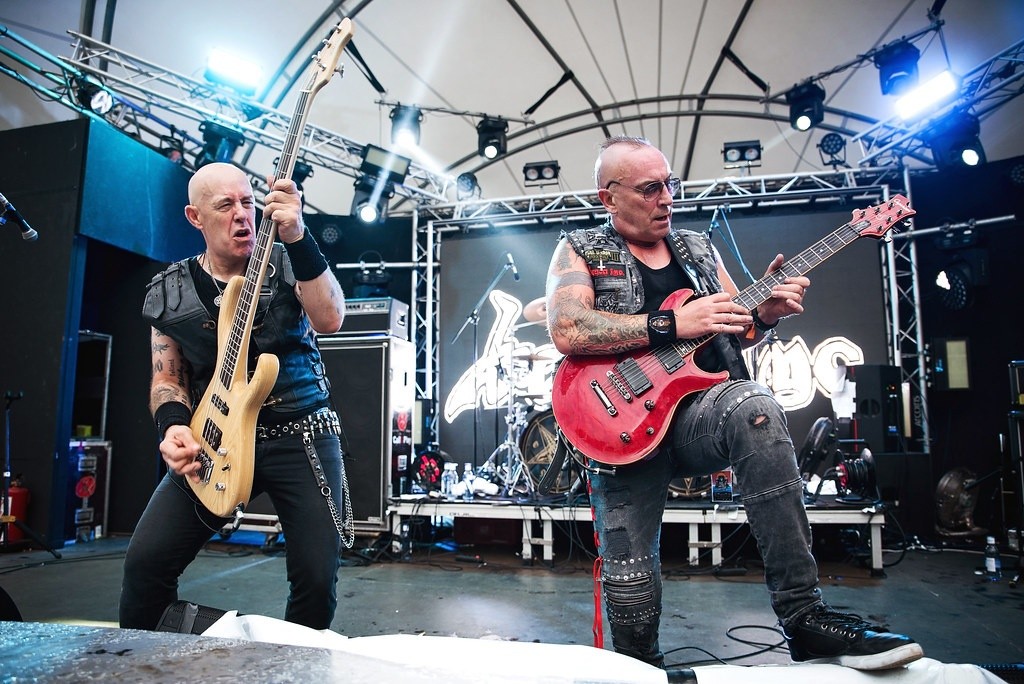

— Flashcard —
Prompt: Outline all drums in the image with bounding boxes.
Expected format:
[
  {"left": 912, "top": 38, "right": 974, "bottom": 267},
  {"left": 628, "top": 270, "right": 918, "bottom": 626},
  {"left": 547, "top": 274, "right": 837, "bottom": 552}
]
[
  {"left": 517, "top": 406, "right": 588, "bottom": 494},
  {"left": 667, "top": 472, "right": 713, "bottom": 497}
]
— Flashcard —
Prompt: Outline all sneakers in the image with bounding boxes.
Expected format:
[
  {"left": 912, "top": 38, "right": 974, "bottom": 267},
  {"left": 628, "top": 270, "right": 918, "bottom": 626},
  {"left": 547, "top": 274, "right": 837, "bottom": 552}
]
[{"left": 784, "top": 600, "right": 924, "bottom": 670}]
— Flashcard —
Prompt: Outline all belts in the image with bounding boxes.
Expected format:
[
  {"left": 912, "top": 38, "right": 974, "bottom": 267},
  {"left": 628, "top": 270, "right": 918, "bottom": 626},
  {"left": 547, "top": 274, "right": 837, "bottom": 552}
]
[{"left": 255, "top": 411, "right": 342, "bottom": 437}]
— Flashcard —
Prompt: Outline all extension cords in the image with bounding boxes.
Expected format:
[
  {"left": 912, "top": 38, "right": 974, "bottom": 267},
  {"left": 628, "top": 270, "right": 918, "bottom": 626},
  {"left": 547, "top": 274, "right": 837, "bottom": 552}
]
[
  {"left": 455, "top": 555, "right": 483, "bottom": 563},
  {"left": 714, "top": 567, "right": 748, "bottom": 577}
]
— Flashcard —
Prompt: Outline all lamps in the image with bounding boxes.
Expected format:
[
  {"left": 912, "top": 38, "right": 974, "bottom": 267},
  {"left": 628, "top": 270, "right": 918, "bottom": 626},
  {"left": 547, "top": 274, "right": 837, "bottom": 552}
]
[
  {"left": 787, "top": 83, "right": 826, "bottom": 131},
  {"left": 477, "top": 116, "right": 507, "bottom": 158},
  {"left": 523, "top": 160, "right": 560, "bottom": 186},
  {"left": 929, "top": 133, "right": 985, "bottom": 171},
  {"left": 274, "top": 152, "right": 313, "bottom": 209},
  {"left": 350, "top": 173, "right": 395, "bottom": 224},
  {"left": 76, "top": 78, "right": 113, "bottom": 115},
  {"left": 194, "top": 120, "right": 245, "bottom": 170},
  {"left": 456, "top": 172, "right": 481, "bottom": 201},
  {"left": 876, "top": 40, "right": 919, "bottom": 93},
  {"left": 390, "top": 106, "right": 423, "bottom": 146},
  {"left": 721, "top": 140, "right": 763, "bottom": 168},
  {"left": 817, "top": 133, "right": 846, "bottom": 174}
]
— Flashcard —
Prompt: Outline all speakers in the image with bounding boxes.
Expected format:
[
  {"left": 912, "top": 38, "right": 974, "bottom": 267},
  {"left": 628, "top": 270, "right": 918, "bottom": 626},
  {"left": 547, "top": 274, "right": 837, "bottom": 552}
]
[
  {"left": 154, "top": 599, "right": 243, "bottom": 637},
  {"left": 219, "top": 336, "right": 417, "bottom": 537},
  {"left": 854, "top": 364, "right": 902, "bottom": 454}
]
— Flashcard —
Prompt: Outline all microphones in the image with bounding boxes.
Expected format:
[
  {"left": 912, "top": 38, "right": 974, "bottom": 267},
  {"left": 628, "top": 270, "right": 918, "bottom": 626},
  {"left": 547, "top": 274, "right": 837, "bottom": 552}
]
[
  {"left": 0, "top": 192, "right": 38, "bottom": 241},
  {"left": 507, "top": 251, "right": 519, "bottom": 282}
]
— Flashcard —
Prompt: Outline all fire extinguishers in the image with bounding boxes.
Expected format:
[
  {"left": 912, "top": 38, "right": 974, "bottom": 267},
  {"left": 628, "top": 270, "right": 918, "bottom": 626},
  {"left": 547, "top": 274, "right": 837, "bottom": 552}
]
[{"left": 0, "top": 468, "right": 30, "bottom": 542}]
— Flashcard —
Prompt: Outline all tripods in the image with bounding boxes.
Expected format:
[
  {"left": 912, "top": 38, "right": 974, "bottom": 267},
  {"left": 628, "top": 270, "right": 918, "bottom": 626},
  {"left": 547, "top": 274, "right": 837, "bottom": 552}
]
[
  {"left": 0, "top": 390, "right": 62, "bottom": 560},
  {"left": 464, "top": 344, "right": 537, "bottom": 503}
]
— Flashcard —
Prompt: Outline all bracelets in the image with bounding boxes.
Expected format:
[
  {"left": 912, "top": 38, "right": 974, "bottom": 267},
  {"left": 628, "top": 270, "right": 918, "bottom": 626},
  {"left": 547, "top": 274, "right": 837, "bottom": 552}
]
[
  {"left": 647, "top": 309, "right": 677, "bottom": 344},
  {"left": 751, "top": 308, "right": 780, "bottom": 331},
  {"left": 153, "top": 401, "right": 192, "bottom": 442},
  {"left": 282, "top": 225, "right": 328, "bottom": 281}
]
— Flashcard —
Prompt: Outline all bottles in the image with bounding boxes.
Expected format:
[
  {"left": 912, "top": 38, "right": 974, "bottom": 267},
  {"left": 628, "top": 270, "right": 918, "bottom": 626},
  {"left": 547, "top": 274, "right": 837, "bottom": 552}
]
[
  {"left": 462, "top": 462, "right": 474, "bottom": 500},
  {"left": 1007, "top": 529, "right": 1019, "bottom": 551},
  {"left": 400, "top": 524, "right": 412, "bottom": 561},
  {"left": 984, "top": 536, "right": 1003, "bottom": 582},
  {"left": 441, "top": 462, "right": 459, "bottom": 500}
]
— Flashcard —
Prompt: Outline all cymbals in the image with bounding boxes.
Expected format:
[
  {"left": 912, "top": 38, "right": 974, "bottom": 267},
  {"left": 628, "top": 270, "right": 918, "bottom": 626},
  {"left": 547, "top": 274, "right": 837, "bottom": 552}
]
[{"left": 513, "top": 354, "right": 553, "bottom": 361}]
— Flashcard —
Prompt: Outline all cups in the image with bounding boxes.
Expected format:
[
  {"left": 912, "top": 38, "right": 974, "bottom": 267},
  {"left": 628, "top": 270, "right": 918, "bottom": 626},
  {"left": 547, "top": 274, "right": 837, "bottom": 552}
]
[{"left": 76, "top": 425, "right": 91, "bottom": 439}]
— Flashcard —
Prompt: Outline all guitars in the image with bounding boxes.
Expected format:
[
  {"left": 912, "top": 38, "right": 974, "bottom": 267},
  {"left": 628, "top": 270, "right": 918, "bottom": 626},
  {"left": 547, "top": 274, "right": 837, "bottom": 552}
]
[
  {"left": 549, "top": 190, "right": 919, "bottom": 468},
  {"left": 181, "top": 14, "right": 354, "bottom": 519}
]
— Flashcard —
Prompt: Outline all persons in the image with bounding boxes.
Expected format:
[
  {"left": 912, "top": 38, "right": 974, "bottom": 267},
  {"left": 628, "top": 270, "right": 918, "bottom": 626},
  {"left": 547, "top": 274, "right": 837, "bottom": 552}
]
[
  {"left": 545, "top": 135, "right": 925, "bottom": 672},
  {"left": 118, "top": 160, "right": 355, "bottom": 635}
]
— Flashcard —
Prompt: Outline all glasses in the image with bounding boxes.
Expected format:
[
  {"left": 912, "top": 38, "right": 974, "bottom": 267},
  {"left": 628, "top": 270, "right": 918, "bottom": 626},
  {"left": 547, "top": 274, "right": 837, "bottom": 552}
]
[{"left": 606, "top": 178, "right": 681, "bottom": 202}]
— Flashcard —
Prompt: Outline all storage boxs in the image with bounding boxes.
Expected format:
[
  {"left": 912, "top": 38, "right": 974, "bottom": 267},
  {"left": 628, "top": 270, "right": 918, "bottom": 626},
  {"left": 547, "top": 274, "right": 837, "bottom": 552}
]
[
  {"left": 220, "top": 336, "right": 416, "bottom": 553},
  {"left": 64, "top": 331, "right": 116, "bottom": 543}
]
